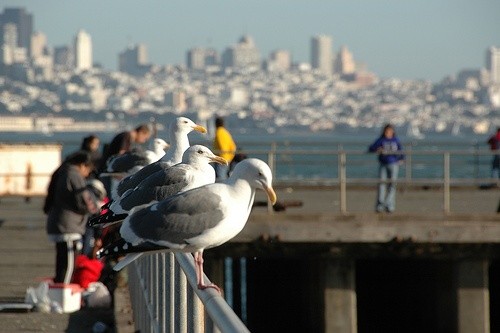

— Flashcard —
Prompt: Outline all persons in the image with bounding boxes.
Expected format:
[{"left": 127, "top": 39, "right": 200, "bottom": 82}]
[
  {"left": 43, "top": 123, "right": 154, "bottom": 283},
  {"left": 487, "top": 128, "right": 500, "bottom": 180},
  {"left": 369, "top": 125, "right": 405, "bottom": 213},
  {"left": 215, "top": 117, "right": 237, "bottom": 180}
]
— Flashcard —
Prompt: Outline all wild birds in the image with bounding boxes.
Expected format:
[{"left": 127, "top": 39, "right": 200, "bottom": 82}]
[
  {"left": 95, "top": 158, "right": 276, "bottom": 294},
  {"left": 85, "top": 144, "right": 229, "bottom": 230},
  {"left": 98, "top": 138, "right": 171, "bottom": 180},
  {"left": 85, "top": 123, "right": 153, "bottom": 180},
  {"left": 100, "top": 116, "right": 207, "bottom": 211}
]
